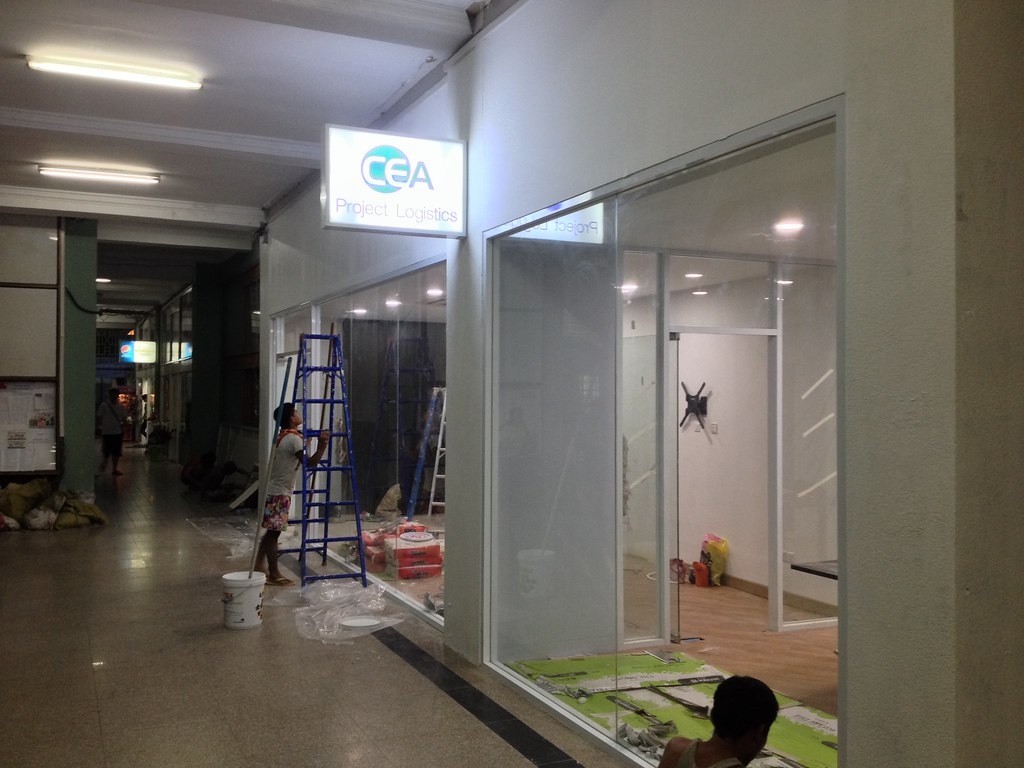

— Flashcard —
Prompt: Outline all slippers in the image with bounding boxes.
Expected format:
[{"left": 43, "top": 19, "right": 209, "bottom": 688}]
[
  {"left": 100, "top": 462, "right": 107, "bottom": 473},
  {"left": 254, "top": 567, "right": 271, "bottom": 578},
  {"left": 112, "top": 470, "right": 124, "bottom": 477},
  {"left": 265, "top": 575, "right": 297, "bottom": 587}
]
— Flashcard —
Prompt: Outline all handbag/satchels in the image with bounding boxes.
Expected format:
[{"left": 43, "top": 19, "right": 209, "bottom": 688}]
[{"left": 700, "top": 533, "right": 728, "bottom": 586}]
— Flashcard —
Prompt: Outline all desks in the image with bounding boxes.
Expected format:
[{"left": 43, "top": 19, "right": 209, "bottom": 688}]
[{"left": 790, "top": 560, "right": 839, "bottom": 580}]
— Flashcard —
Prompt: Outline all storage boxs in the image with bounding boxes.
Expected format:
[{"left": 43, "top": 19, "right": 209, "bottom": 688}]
[
  {"left": 383, "top": 522, "right": 442, "bottom": 580},
  {"left": 148, "top": 444, "right": 168, "bottom": 461}
]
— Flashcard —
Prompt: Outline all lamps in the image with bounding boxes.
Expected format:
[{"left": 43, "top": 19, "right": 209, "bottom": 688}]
[
  {"left": 37, "top": 164, "right": 160, "bottom": 185},
  {"left": 26, "top": 61, "right": 203, "bottom": 90}
]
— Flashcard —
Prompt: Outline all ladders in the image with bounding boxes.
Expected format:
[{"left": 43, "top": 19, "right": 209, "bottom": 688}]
[
  {"left": 273, "top": 332, "right": 367, "bottom": 587},
  {"left": 366, "top": 334, "right": 445, "bottom": 517},
  {"left": 408, "top": 384, "right": 446, "bottom": 519}
]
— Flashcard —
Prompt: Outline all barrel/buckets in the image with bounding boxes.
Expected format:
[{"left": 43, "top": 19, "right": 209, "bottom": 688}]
[{"left": 222, "top": 572, "right": 267, "bottom": 629}]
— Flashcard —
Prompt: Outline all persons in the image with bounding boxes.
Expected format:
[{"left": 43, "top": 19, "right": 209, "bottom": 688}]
[
  {"left": 657, "top": 673, "right": 780, "bottom": 768},
  {"left": 96, "top": 388, "right": 128, "bottom": 476},
  {"left": 253, "top": 402, "right": 330, "bottom": 587},
  {"left": 135, "top": 394, "right": 148, "bottom": 442}
]
